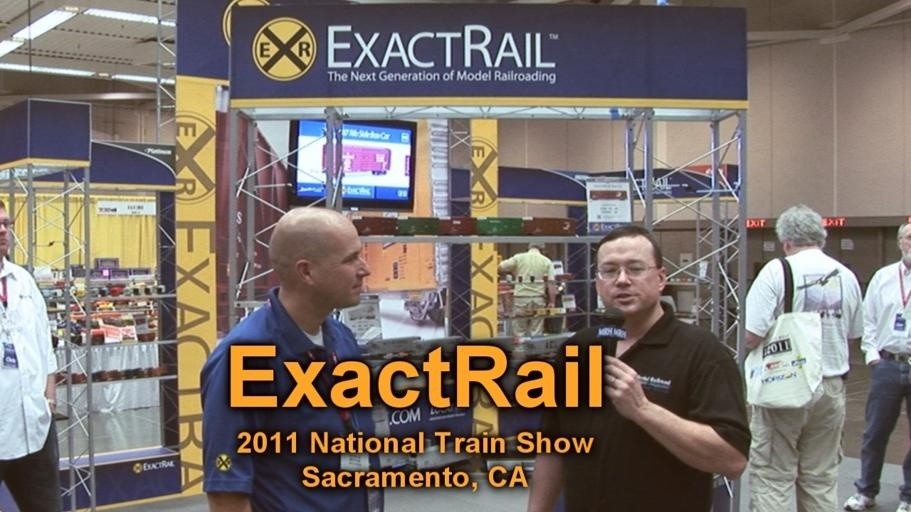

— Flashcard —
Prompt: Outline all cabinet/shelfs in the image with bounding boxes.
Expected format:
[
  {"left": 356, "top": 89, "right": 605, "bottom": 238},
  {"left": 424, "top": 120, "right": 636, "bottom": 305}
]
[{"left": 21, "top": 265, "right": 160, "bottom": 383}]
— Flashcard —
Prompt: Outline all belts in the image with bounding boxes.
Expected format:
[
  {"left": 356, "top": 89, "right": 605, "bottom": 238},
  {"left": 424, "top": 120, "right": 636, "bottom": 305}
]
[{"left": 879, "top": 349, "right": 911, "bottom": 362}]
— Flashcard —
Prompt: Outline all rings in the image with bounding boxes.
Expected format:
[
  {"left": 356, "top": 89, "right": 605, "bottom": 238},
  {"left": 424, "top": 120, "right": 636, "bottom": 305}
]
[{"left": 608, "top": 376, "right": 616, "bottom": 386}]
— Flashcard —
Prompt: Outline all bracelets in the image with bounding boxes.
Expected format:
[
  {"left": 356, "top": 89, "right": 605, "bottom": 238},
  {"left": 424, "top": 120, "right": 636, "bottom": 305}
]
[{"left": 48, "top": 398, "right": 57, "bottom": 406}]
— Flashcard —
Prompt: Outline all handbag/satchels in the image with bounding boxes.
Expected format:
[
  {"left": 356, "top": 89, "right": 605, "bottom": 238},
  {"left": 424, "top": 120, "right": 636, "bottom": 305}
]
[{"left": 742, "top": 309, "right": 824, "bottom": 410}]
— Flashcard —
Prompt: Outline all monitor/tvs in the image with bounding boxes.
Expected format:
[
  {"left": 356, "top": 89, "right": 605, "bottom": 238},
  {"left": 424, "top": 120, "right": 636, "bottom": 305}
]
[{"left": 290, "top": 119, "right": 417, "bottom": 213}]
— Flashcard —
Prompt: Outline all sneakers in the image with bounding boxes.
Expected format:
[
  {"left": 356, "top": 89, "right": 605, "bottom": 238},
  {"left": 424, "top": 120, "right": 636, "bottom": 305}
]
[
  {"left": 843, "top": 492, "right": 874, "bottom": 512},
  {"left": 895, "top": 501, "right": 911, "bottom": 512}
]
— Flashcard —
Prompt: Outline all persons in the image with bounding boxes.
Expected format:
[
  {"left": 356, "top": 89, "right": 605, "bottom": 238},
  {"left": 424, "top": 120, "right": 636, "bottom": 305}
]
[
  {"left": 527, "top": 226, "right": 751, "bottom": 512},
  {"left": 199, "top": 206, "right": 386, "bottom": 512},
  {"left": 498, "top": 239, "right": 556, "bottom": 337},
  {"left": 843, "top": 222, "right": 911, "bottom": 512},
  {"left": 0, "top": 201, "right": 63, "bottom": 512},
  {"left": 744, "top": 205, "right": 864, "bottom": 512}
]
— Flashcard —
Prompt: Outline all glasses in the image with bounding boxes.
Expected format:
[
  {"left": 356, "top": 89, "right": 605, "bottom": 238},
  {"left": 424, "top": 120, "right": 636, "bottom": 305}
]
[
  {"left": 0, "top": 218, "right": 15, "bottom": 228},
  {"left": 593, "top": 259, "right": 666, "bottom": 283}
]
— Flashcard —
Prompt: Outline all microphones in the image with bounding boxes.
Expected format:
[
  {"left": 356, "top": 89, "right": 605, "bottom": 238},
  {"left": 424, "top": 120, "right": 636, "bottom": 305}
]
[{"left": 598, "top": 307, "right": 627, "bottom": 421}]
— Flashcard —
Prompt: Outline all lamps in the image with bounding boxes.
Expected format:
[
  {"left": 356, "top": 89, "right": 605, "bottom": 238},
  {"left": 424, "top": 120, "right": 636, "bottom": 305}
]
[
  {"left": 82, "top": 8, "right": 178, "bottom": 29},
  {"left": 11, "top": 5, "right": 79, "bottom": 37},
  {"left": 0, "top": 40, "right": 24, "bottom": 58}
]
